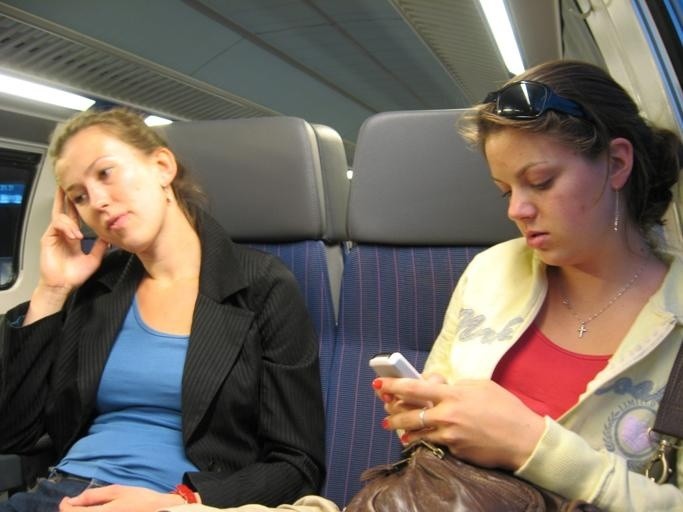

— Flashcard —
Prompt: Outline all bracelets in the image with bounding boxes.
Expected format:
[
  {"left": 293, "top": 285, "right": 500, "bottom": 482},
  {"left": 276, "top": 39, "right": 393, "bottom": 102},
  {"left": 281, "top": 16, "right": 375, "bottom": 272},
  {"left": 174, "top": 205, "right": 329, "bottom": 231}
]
[{"left": 167, "top": 483, "right": 199, "bottom": 505}]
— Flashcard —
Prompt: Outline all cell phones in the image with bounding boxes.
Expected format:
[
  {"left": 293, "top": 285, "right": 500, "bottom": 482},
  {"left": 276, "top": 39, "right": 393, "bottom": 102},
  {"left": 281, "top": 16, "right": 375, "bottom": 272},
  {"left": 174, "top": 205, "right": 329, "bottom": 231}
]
[{"left": 369, "top": 352, "right": 435, "bottom": 409}]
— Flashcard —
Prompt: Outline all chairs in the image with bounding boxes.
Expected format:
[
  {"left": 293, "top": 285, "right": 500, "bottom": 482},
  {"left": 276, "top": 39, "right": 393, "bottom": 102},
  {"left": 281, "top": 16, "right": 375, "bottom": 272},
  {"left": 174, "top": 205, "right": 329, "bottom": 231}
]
[{"left": 323, "top": 108, "right": 528, "bottom": 512}]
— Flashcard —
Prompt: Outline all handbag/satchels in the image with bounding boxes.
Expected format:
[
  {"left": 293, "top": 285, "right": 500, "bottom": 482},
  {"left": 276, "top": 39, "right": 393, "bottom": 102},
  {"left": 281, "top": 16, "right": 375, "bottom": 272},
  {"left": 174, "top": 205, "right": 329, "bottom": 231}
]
[{"left": 344, "top": 438, "right": 603, "bottom": 512}]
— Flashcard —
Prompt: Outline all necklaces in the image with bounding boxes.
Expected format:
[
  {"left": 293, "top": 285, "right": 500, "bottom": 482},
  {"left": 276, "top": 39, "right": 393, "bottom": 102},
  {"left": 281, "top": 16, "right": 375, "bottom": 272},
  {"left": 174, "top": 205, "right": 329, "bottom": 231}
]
[{"left": 557, "top": 243, "right": 655, "bottom": 342}]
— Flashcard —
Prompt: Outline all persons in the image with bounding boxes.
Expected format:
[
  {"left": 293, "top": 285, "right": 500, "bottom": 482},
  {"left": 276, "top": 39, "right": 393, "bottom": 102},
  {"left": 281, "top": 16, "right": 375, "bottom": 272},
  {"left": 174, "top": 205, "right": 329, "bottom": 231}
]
[{"left": 371, "top": 57, "right": 682, "bottom": 512}]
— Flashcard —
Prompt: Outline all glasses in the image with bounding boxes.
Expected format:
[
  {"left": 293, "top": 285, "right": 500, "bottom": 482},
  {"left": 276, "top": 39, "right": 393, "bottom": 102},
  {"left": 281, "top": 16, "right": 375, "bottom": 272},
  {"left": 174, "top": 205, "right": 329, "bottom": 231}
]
[{"left": 481, "top": 80, "right": 596, "bottom": 124}]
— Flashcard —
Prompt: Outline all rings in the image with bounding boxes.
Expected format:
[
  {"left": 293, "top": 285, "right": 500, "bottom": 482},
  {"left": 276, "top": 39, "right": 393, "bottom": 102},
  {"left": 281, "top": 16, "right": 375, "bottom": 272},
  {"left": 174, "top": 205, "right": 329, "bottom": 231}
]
[{"left": 419, "top": 407, "right": 429, "bottom": 431}]
